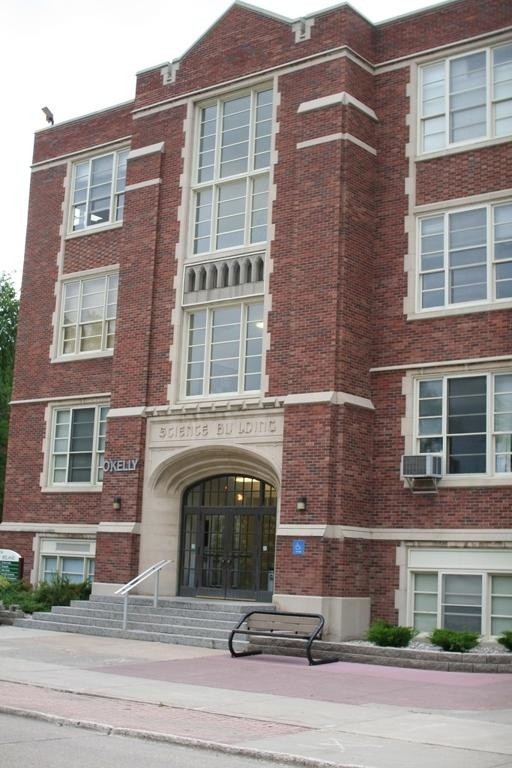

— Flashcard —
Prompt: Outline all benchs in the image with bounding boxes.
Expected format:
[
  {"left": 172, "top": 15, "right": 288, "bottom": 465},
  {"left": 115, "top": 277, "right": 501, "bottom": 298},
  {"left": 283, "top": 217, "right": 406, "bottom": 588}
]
[{"left": 227, "top": 610, "right": 339, "bottom": 666}]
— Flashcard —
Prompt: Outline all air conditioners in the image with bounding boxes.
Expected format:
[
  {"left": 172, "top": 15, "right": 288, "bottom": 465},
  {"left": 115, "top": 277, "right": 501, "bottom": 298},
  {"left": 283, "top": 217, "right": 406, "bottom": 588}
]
[{"left": 400, "top": 453, "right": 442, "bottom": 479}]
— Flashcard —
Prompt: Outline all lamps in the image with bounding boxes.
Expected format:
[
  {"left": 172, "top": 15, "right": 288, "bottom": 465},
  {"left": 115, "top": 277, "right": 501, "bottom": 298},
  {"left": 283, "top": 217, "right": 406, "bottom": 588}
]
[
  {"left": 113, "top": 495, "right": 121, "bottom": 511},
  {"left": 296, "top": 497, "right": 306, "bottom": 511}
]
